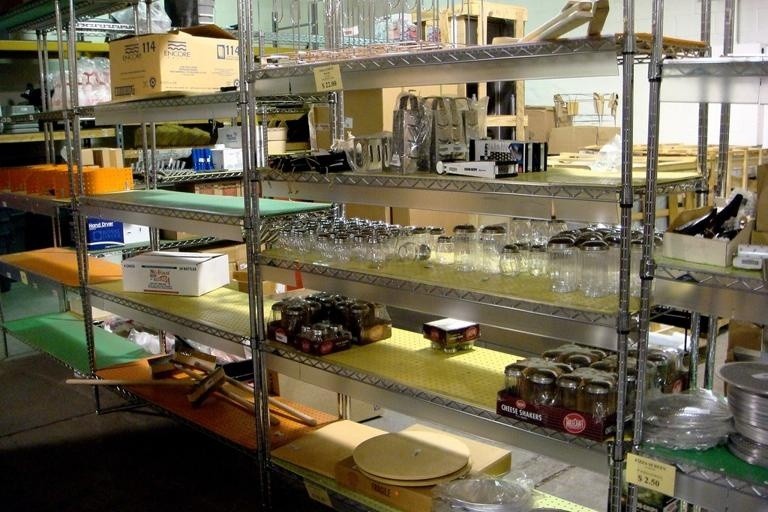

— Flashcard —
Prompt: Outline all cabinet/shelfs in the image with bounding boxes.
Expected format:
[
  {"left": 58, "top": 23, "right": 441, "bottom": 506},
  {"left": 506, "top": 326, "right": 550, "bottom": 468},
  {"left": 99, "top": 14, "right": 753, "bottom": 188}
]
[{"left": 3, "top": 0, "right": 768, "bottom": 512}]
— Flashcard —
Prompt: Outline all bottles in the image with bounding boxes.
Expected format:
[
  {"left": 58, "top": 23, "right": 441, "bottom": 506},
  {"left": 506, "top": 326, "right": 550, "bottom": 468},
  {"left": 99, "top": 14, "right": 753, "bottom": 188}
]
[
  {"left": 279, "top": 216, "right": 645, "bottom": 302},
  {"left": 272, "top": 294, "right": 370, "bottom": 343},
  {"left": 502, "top": 340, "right": 669, "bottom": 421}
]
